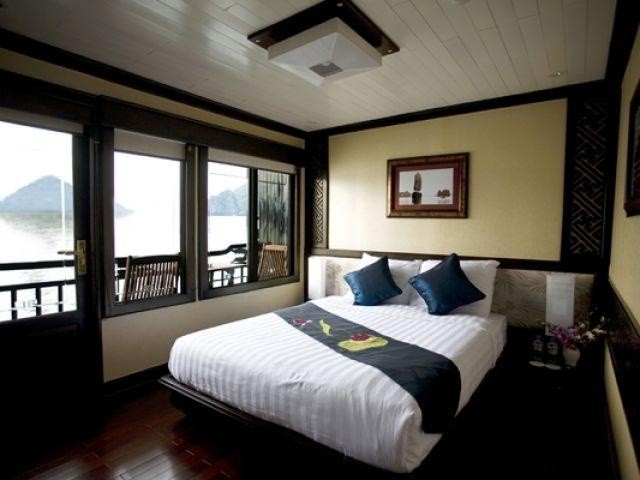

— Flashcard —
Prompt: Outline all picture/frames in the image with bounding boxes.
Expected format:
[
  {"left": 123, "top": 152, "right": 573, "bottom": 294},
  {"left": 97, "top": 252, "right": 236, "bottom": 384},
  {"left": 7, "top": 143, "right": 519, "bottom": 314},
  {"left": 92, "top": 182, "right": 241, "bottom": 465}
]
[{"left": 385, "top": 151, "right": 469, "bottom": 219}]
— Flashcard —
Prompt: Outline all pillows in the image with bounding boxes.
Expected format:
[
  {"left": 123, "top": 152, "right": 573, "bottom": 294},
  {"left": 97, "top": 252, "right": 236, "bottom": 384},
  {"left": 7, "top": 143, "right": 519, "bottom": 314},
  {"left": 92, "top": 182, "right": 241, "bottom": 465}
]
[{"left": 342, "top": 251, "right": 501, "bottom": 320}]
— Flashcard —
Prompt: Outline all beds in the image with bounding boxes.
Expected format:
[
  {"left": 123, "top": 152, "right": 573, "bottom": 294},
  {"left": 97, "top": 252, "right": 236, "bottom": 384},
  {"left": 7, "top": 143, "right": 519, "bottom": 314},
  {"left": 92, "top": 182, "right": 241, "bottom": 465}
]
[{"left": 156, "top": 292, "right": 510, "bottom": 479}]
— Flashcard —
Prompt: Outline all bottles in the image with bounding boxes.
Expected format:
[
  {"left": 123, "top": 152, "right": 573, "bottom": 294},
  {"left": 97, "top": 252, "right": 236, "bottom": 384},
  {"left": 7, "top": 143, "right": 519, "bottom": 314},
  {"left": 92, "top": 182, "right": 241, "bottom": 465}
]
[
  {"left": 531, "top": 336, "right": 544, "bottom": 365},
  {"left": 546, "top": 337, "right": 559, "bottom": 367}
]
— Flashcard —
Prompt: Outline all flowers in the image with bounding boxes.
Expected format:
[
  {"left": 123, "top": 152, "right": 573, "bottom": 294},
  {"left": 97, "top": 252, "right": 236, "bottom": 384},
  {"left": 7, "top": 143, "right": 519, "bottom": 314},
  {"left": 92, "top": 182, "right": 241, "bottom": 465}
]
[{"left": 535, "top": 311, "right": 608, "bottom": 347}]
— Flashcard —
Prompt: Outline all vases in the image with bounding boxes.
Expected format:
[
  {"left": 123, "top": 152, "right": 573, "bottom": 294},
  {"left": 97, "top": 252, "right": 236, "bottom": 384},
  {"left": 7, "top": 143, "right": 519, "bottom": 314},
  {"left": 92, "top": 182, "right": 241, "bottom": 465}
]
[{"left": 563, "top": 343, "right": 581, "bottom": 368}]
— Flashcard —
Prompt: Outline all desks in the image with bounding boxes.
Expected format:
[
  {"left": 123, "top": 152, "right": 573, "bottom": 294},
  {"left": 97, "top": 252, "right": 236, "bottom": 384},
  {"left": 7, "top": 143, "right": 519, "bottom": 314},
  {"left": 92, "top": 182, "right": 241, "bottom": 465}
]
[{"left": 208, "top": 263, "right": 248, "bottom": 288}]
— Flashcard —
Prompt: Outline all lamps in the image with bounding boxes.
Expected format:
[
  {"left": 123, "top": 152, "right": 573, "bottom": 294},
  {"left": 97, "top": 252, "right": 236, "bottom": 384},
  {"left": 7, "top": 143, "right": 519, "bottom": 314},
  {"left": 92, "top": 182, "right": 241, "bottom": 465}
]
[{"left": 246, "top": 0, "right": 401, "bottom": 92}]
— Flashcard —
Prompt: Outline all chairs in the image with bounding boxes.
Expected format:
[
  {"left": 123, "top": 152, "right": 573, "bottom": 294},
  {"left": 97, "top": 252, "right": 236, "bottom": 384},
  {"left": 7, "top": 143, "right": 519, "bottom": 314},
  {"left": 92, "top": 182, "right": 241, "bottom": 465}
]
[
  {"left": 258, "top": 243, "right": 288, "bottom": 279},
  {"left": 121, "top": 252, "right": 180, "bottom": 301}
]
